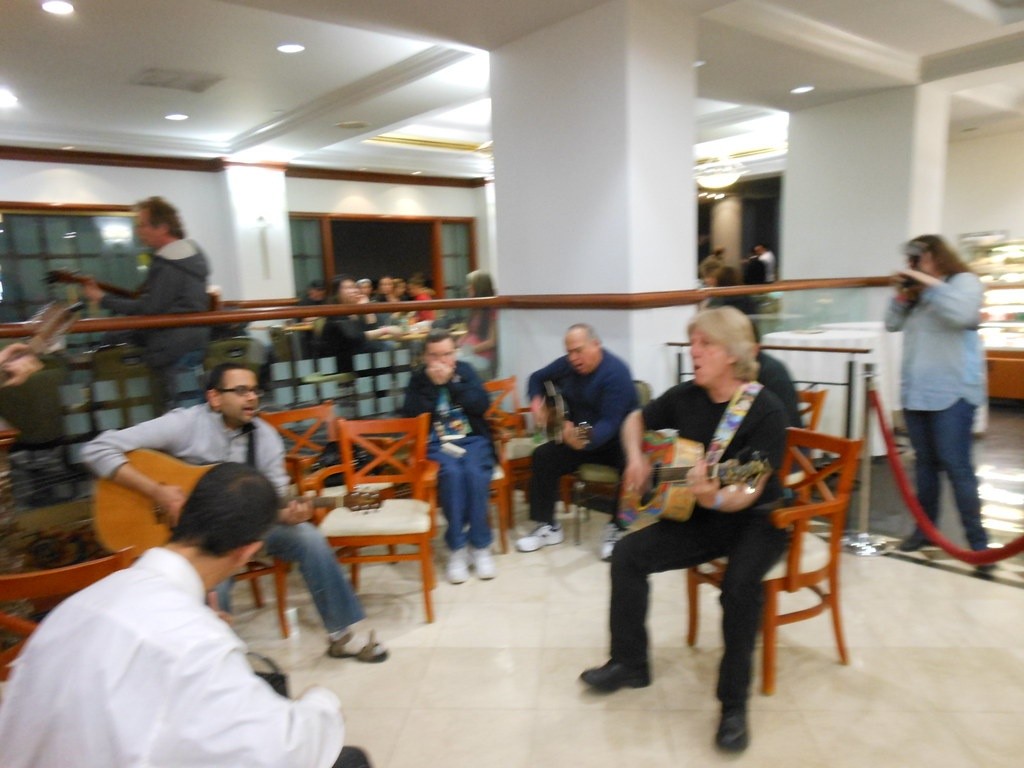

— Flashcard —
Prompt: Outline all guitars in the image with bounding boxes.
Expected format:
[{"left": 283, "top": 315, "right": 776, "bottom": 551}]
[
  {"left": 543, "top": 380, "right": 593, "bottom": 446},
  {"left": 91, "top": 445, "right": 384, "bottom": 561},
  {"left": 617, "top": 436, "right": 771, "bottom": 532}
]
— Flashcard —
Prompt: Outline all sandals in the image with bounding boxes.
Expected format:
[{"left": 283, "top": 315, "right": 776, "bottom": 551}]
[{"left": 326, "top": 630, "right": 388, "bottom": 664}]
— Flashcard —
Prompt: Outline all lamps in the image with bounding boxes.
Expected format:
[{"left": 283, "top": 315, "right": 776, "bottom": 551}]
[{"left": 696, "top": 156, "right": 748, "bottom": 189}]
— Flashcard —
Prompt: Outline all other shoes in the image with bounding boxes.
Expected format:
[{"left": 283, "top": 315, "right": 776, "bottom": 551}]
[
  {"left": 898, "top": 530, "right": 936, "bottom": 551},
  {"left": 448, "top": 548, "right": 469, "bottom": 583},
  {"left": 472, "top": 547, "right": 496, "bottom": 579},
  {"left": 580, "top": 658, "right": 649, "bottom": 690},
  {"left": 716, "top": 698, "right": 749, "bottom": 749}
]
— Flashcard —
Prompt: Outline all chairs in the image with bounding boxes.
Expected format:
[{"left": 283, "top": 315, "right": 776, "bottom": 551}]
[
  {"left": 783, "top": 387, "right": 827, "bottom": 498},
  {"left": 293, "top": 409, "right": 443, "bottom": 627},
  {"left": 573, "top": 378, "right": 661, "bottom": 547},
  {"left": 415, "top": 418, "right": 512, "bottom": 557},
  {"left": 0, "top": 544, "right": 139, "bottom": 681},
  {"left": 685, "top": 427, "right": 866, "bottom": 701},
  {"left": 484, "top": 375, "right": 576, "bottom": 530},
  {"left": 257, "top": 400, "right": 396, "bottom": 588},
  {"left": 226, "top": 546, "right": 291, "bottom": 639}
]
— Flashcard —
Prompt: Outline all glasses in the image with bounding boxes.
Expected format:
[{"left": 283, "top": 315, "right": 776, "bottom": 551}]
[{"left": 220, "top": 384, "right": 264, "bottom": 398}]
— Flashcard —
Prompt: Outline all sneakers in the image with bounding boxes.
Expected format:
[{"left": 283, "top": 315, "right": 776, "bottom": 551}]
[
  {"left": 516, "top": 524, "right": 564, "bottom": 551},
  {"left": 601, "top": 522, "right": 631, "bottom": 558}
]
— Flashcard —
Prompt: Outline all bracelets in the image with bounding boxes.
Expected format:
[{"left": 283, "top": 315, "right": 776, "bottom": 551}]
[{"left": 709, "top": 493, "right": 723, "bottom": 510}]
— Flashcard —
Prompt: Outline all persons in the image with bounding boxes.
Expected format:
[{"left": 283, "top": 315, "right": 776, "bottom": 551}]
[
  {"left": 455, "top": 269, "right": 498, "bottom": 372},
  {"left": 0, "top": 343, "right": 75, "bottom": 567},
  {"left": 885, "top": 233, "right": 999, "bottom": 572},
  {"left": 755, "top": 245, "right": 778, "bottom": 284},
  {"left": 79, "top": 361, "right": 390, "bottom": 664},
  {"left": 0, "top": 461, "right": 372, "bottom": 768},
  {"left": 401, "top": 330, "right": 499, "bottom": 584},
  {"left": 514, "top": 323, "right": 641, "bottom": 560},
  {"left": 751, "top": 320, "right": 809, "bottom": 457},
  {"left": 578, "top": 305, "right": 788, "bottom": 752},
  {"left": 700, "top": 246, "right": 762, "bottom": 314},
  {"left": 317, "top": 272, "right": 438, "bottom": 355},
  {"left": 81, "top": 194, "right": 209, "bottom": 420},
  {"left": 289, "top": 279, "right": 331, "bottom": 321}
]
